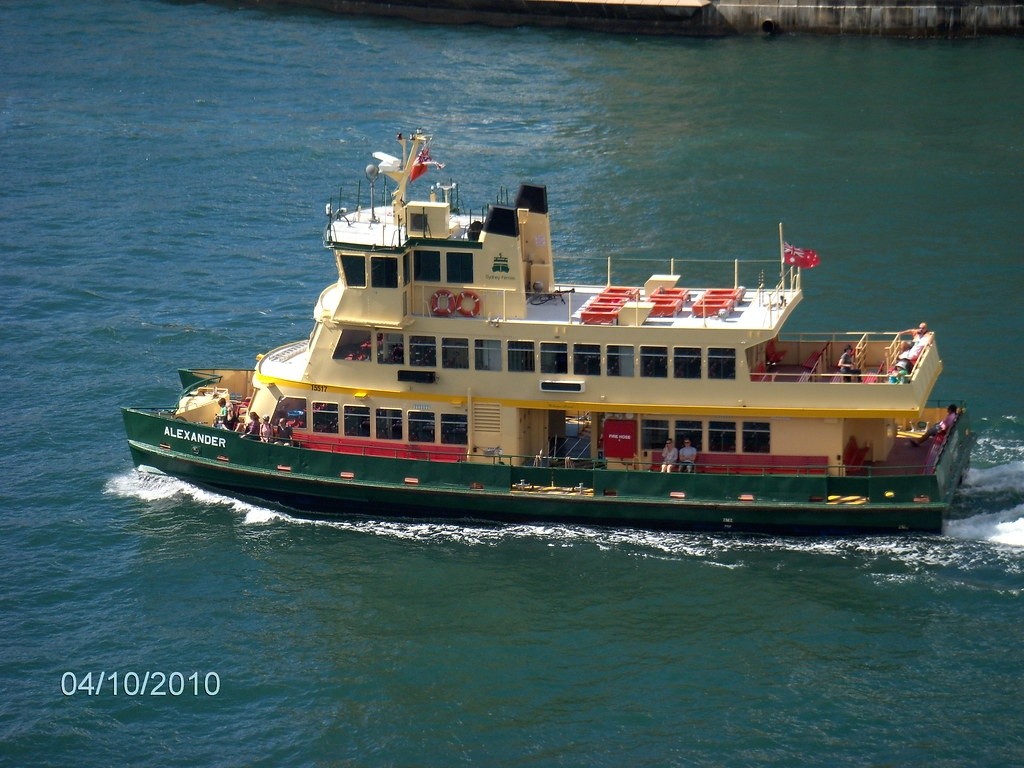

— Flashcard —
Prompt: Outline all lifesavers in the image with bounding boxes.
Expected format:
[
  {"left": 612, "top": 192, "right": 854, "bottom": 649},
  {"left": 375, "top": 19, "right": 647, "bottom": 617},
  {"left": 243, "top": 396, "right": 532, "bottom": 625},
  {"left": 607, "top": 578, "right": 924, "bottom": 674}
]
[
  {"left": 455, "top": 290, "right": 481, "bottom": 318},
  {"left": 429, "top": 289, "right": 456, "bottom": 315}
]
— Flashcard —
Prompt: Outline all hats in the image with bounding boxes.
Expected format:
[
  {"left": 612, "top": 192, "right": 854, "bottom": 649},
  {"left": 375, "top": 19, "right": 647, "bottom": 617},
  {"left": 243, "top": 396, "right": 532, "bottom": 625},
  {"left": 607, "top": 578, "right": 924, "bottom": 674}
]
[
  {"left": 844, "top": 345, "right": 852, "bottom": 352},
  {"left": 263, "top": 415, "right": 269, "bottom": 420},
  {"left": 280, "top": 418, "right": 285, "bottom": 423},
  {"left": 896, "top": 361, "right": 907, "bottom": 369}
]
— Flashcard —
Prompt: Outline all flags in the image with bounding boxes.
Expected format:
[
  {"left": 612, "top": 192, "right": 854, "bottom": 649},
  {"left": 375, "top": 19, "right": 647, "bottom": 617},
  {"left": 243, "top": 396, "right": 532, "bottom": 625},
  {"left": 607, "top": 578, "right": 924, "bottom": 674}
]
[
  {"left": 409, "top": 147, "right": 431, "bottom": 178},
  {"left": 783, "top": 244, "right": 819, "bottom": 268}
]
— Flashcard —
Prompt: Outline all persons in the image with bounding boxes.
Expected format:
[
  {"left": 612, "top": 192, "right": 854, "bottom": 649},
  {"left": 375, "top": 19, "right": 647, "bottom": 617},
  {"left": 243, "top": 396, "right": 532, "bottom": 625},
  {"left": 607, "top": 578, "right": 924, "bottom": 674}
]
[
  {"left": 242, "top": 412, "right": 261, "bottom": 439},
  {"left": 212, "top": 399, "right": 227, "bottom": 426},
  {"left": 259, "top": 416, "right": 273, "bottom": 443},
  {"left": 219, "top": 407, "right": 240, "bottom": 432},
  {"left": 838, "top": 344, "right": 862, "bottom": 382},
  {"left": 661, "top": 439, "right": 678, "bottom": 473},
  {"left": 273, "top": 418, "right": 293, "bottom": 447},
  {"left": 909, "top": 404, "right": 957, "bottom": 445},
  {"left": 228, "top": 401, "right": 247, "bottom": 421},
  {"left": 678, "top": 438, "right": 697, "bottom": 473},
  {"left": 891, "top": 323, "right": 932, "bottom": 383}
]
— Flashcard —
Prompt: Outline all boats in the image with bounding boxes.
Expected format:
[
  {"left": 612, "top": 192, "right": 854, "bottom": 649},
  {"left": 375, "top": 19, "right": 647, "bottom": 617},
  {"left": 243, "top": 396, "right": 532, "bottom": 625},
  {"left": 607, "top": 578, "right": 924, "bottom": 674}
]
[{"left": 122, "top": 131, "right": 978, "bottom": 538}]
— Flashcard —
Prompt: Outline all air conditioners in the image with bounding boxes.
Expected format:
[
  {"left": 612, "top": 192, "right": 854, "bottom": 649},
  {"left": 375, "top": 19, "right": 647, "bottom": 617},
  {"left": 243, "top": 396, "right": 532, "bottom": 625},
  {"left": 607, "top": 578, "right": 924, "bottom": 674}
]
[{"left": 407, "top": 201, "right": 450, "bottom": 238}]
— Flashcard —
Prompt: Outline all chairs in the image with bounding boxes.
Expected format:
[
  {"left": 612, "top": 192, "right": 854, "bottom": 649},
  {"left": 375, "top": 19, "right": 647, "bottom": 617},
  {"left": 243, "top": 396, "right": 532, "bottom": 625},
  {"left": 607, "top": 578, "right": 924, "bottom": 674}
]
[
  {"left": 751, "top": 340, "right": 787, "bottom": 383},
  {"left": 792, "top": 341, "right": 884, "bottom": 383}
]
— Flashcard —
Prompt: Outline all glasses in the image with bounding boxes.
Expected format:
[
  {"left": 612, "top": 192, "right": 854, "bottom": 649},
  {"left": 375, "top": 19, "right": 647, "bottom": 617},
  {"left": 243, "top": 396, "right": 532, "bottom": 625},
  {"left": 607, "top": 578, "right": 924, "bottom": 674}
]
[
  {"left": 684, "top": 442, "right": 689, "bottom": 444},
  {"left": 666, "top": 443, "right": 671, "bottom": 444}
]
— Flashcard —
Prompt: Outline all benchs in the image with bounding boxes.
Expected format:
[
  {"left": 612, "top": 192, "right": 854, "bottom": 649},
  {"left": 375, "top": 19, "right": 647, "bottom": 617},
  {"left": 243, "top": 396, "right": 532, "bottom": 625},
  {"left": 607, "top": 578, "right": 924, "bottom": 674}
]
[
  {"left": 273, "top": 428, "right": 468, "bottom": 463},
  {"left": 842, "top": 436, "right": 874, "bottom": 475},
  {"left": 649, "top": 451, "right": 829, "bottom": 476},
  {"left": 915, "top": 410, "right": 962, "bottom": 475}
]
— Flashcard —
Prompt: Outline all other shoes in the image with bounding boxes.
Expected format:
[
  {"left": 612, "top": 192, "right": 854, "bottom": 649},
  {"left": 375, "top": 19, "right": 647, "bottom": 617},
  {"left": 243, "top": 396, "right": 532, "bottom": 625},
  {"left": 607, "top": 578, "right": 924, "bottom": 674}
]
[{"left": 909, "top": 439, "right": 919, "bottom": 448}]
